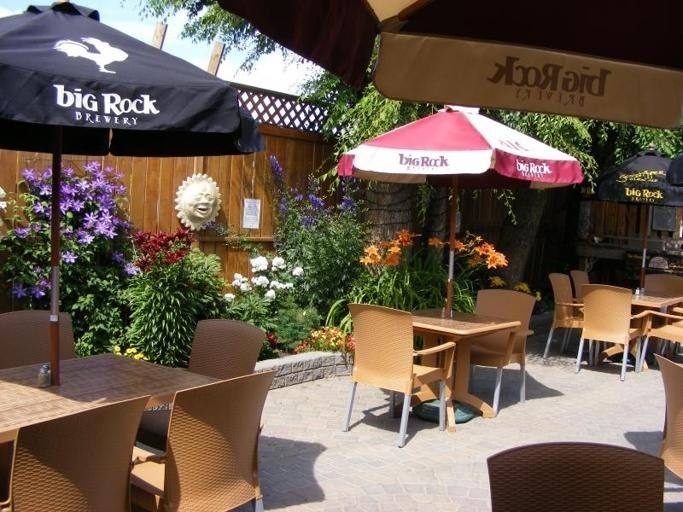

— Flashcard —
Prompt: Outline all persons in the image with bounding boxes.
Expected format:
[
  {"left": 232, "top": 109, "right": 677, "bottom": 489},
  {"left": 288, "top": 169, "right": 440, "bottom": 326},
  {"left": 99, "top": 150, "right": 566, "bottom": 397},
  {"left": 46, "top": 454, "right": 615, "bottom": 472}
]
[{"left": 182, "top": 180, "right": 217, "bottom": 222}]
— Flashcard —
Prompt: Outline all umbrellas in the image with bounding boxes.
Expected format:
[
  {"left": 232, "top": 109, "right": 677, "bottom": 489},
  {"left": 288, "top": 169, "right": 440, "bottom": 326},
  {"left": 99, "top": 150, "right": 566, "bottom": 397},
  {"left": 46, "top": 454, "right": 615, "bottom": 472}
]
[
  {"left": 579, "top": 142, "right": 682, "bottom": 289},
  {"left": 200, "top": 0, "right": 680, "bottom": 129},
  {"left": 0, "top": 0, "right": 266, "bottom": 384},
  {"left": 337, "top": 104, "right": 585, "bottom": 320},
  {"left": 667, "top": 156, "right": 683, "bottom": 186}
]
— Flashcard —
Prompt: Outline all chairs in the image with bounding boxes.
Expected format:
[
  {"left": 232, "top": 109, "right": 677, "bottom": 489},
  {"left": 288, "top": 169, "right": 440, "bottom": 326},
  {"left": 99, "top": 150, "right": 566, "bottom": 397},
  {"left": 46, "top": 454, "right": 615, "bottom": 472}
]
[
  {"left": 640, "top": 305, "right": 682, "bottom": 373},
  {"left": 652, "top": 352, "right": 683, "bottom": 482},
  {"left": 1, "top": 394, "right": 153, "bottom": 510},
  {"left": 445, "top": 289, "right": 538, "bottom": 417},
  {"left": 643, "top": 274, "right": 683, "bottom": 299},
  {"left": 575, "top": 283, "right": 648, "bottom": 382},
  {"left": 486, "top": 440, "right": 665, "bottom": 511},
  {"left": 1, "top": 310, "right": 77, "bottom": 368},
  {"left": 347, "top": 302, "right": 457, "bottom": 449},
  {"left": 564, "top": 271, "right": 592, "bottom": 354},
  {"left": 542, "top": 273, "right": 594, "bottom": 368},
  {"left": 134, "top": 319, "right": 267, "bottom": 457},
  {"left": 129, "top": 371, "right": 275, "bottom": 511}
]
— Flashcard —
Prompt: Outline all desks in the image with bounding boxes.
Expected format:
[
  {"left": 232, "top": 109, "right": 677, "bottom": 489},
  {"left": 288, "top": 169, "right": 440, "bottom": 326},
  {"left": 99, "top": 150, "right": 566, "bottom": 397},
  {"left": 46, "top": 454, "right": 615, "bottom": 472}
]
[
  {"left": 392, "top": 307, "right": 521, "bottom": 419},
  {"left": 581, "top": 290, "right": 683, "bottom": 373},
  {"left": 648, "top": 322, "right": 683, "bottom": 345},
  {"left": 2, "top": 353, "right": 223, "bottom": 448}
]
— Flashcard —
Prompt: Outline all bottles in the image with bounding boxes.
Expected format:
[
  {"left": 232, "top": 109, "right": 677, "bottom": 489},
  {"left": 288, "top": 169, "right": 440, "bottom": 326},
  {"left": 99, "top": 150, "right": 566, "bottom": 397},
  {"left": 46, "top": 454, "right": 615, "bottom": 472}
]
[
  {"left": 36, "top": 364, "right": 51, "bottom": 388},
  {"left": 440, "top": 307, "right": 445, "bottom": 318},
  {"left": 636, "top": 287, "right": 640, "bottom": 294}
]
